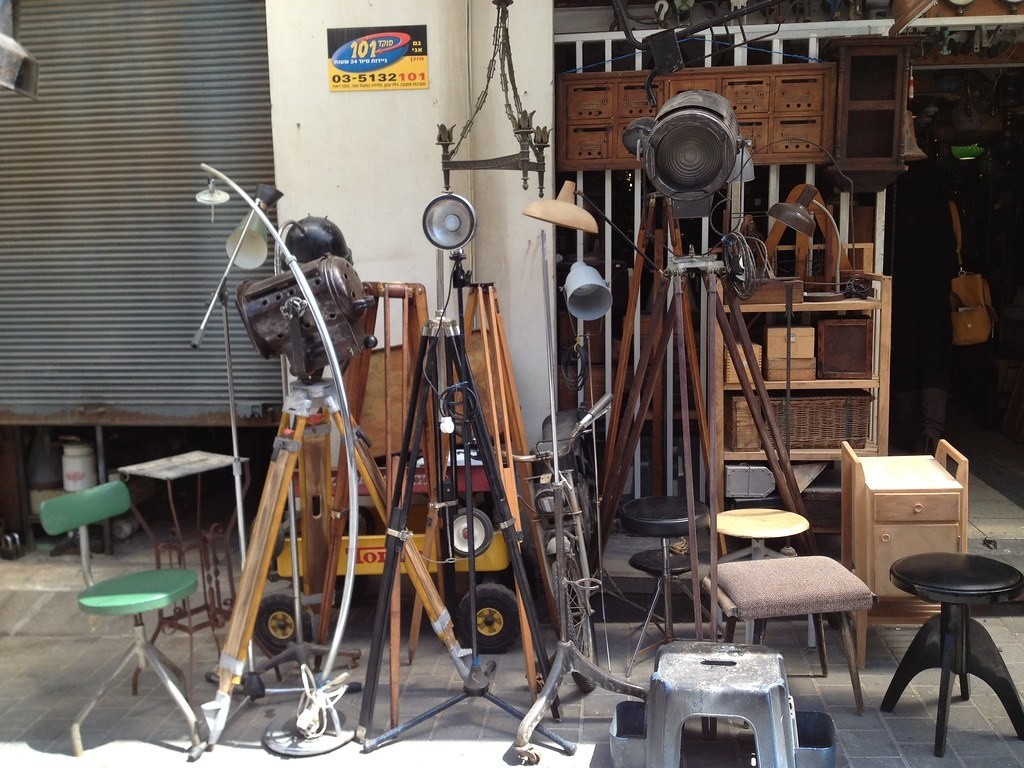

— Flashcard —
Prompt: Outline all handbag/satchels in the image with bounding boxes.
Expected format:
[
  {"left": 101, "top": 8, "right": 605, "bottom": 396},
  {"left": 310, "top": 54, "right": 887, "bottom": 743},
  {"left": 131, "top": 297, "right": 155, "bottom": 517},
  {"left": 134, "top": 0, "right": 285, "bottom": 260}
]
[{"left": 949, "top": 271, "right": 997, "bottom": 346}]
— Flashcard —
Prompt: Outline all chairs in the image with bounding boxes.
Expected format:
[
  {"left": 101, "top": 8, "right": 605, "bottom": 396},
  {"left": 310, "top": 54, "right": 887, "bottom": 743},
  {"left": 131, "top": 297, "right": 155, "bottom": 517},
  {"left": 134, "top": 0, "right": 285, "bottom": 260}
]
[{"left": 41, "top": 481, "right": 202, "bottom": 758}]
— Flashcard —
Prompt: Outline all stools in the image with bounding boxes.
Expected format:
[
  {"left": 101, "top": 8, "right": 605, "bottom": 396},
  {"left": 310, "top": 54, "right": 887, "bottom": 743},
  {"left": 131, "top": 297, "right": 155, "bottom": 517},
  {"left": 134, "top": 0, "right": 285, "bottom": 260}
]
[
  {"left": 880, "top": 553, "right": 1024, "bottom": 757},
  {"left": 621, "top": 495, "right": 726, "bottom": 678},
  {"left": 702, "top": 556, "right": 879, "bottom": 716},
  {"left": 644, "top": 640, "right": 799, "bottom": 768},
  {"left": 709, "top": 508, "right": 817, "bottom": 648}
]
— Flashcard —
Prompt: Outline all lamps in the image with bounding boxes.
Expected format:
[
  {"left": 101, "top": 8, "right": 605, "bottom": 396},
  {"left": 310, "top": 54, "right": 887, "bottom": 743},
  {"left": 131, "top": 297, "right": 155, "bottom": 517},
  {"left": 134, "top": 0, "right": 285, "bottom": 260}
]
[
  {"left": 612, "top": 0, "right": 938, "bottom": 107},
  {"left": 622, "top": 89, "right": 754, "bottom": 641},
  {"left": 766, "top": 186, "right": 845, "bottom": 302},
  {"left": 565, "top": 262, "right": 613, "bottom": 411},
  {"left": 521, "top": 181, "right": 667, "bottom": 279},
  {"left": 254, "top": 213, "right": 362, "bottom": 677},
  {"left": 188, "top": 184, "right": 363, "bottom": 761},
  {"left": 363, "top": 195, "right": 575, "bottom": 755},
  {"left": 700, "top": 148, "right": 755, "bottom": 501}
]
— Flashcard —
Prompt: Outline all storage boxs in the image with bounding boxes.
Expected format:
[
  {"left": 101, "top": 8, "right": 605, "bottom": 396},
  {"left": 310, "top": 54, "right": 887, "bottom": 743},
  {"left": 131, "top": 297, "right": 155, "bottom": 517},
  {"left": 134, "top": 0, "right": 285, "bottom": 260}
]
[
  {"left": 725, "top": 281, "right": 804, "bottom": 304},
  {"left": 724, "top": 395, "right": 762, "bottom": 452},
  {"left": 559, "top": 310, "right": 699, "bottom": 413},
  {"left": 802, "top": 470, "right": 841, "bottom": 528},
  {"left": 723, "top": 314, "right": 873, "bottom": 383}
]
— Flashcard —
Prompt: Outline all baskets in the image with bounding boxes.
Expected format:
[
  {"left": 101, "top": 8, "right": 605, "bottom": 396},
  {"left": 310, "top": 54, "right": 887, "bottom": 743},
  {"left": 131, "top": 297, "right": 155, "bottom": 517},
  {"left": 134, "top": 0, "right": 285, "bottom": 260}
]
[{"left": 760, "top": 388, "right": 875, "bottom": 450}]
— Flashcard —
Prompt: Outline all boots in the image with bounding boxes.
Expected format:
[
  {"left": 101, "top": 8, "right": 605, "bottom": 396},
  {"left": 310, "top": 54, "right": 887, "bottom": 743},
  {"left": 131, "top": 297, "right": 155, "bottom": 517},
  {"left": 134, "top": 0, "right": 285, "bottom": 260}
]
[
  {"left": 893, "top": 389, "right": 919, "bottom": 452},
  {"left": 917, "top": 388, "right": 946, "bottom": 453}
]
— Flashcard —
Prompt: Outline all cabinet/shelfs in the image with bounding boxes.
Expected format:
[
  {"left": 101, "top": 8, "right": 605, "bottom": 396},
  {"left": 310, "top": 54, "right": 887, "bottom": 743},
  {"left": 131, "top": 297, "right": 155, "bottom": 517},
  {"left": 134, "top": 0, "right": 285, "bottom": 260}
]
[
  {"left": 841, "top": 439, "right": 969, "bottom": 626},
  {"left": 713, "top": 273, "right": 892, "bottom": 555},
  {"left": 556, "top": 63, "right": 838, "bottom": 172},
  {"left": 820, "top": 34, "right": 927, "bottom": 194}
]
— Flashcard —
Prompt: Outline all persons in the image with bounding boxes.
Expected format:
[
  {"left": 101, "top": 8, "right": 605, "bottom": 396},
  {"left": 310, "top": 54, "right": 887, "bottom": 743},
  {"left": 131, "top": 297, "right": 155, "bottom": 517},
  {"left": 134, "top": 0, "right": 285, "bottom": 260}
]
[{"left": 892, "top": 173, "right": 974, "bottom": 456}]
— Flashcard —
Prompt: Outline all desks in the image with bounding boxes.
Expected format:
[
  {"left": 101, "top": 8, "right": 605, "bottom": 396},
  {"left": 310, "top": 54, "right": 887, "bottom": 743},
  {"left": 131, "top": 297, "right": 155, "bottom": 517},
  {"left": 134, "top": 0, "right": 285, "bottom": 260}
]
[{"left": 117, "top": 450, "right": 250, "bottom": 696}]
